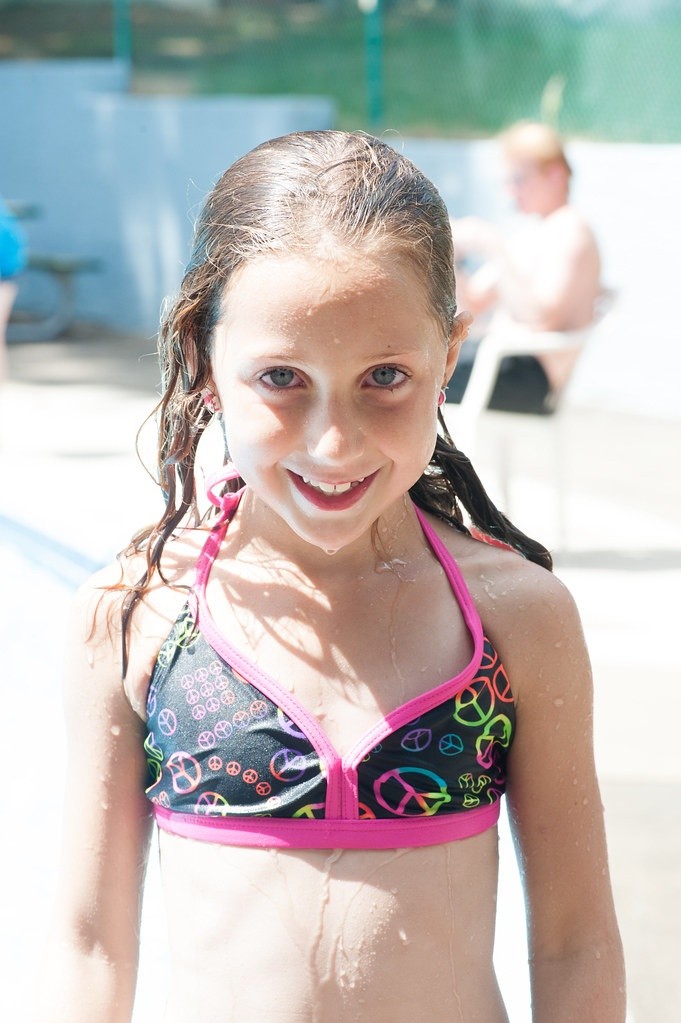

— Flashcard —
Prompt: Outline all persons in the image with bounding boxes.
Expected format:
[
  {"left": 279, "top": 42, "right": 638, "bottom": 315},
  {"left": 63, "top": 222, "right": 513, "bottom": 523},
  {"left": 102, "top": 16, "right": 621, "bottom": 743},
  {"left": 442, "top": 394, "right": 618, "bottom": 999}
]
[
  {"left": 50, "top": 128, "right": 629, "bottom": 1023},
  {"left": 438, "top": 120, "right": 601, "bottom": 416},
  {"left": 0, "top": 194, "right": 27, "bottom": 384}
]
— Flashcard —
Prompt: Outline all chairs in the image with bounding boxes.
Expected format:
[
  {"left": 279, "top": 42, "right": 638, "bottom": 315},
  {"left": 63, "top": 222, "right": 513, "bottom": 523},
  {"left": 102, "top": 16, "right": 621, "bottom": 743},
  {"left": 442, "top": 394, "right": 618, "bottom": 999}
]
[{"left": 431, "top": 285, "right": 620, "bottom": 547}]
